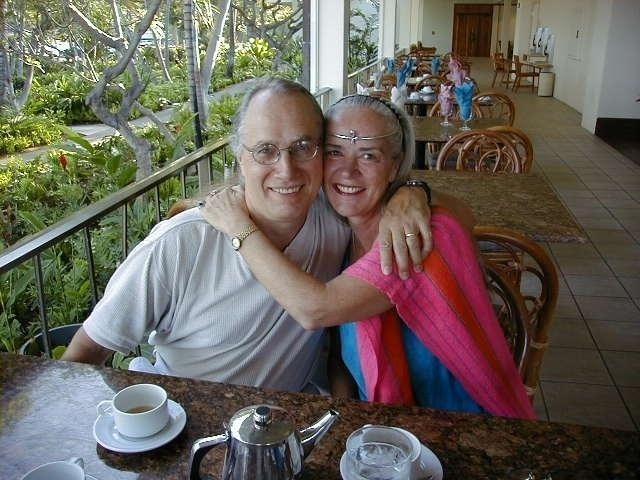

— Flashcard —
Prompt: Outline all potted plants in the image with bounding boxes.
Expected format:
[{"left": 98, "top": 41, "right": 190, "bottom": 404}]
[{"left": 16, "top": 323, "right": 139, "bottom": 371}]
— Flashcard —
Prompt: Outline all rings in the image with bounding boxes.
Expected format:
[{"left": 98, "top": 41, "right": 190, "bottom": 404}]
[{"left": 405, "top": 232, "right": 418, "bottom": 238}]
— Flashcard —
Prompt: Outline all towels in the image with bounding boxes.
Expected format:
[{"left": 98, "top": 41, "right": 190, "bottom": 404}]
[{"left": 356, "top": 82, "right": 363, "bottom": 95}]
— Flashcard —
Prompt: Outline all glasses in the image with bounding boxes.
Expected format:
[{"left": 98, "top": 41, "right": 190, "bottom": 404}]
[{"left": 241, "top": 139, "right": 322, "bottom": 164}]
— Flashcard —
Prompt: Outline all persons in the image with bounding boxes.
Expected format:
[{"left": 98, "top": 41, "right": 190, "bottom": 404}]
[
  {"left": 198, "top": 92, "right": 538, "bottom": 421},
  {"left": 57, "top": 78, "right": 478, "bottom": 393}
]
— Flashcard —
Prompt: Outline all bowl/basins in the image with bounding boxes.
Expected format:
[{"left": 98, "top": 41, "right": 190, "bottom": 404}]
[
  {"left": 410, "top": 83, "right": 434, "bottom": 100},
  {"left": 363, "top": 422, "right": 422, "bottom": 463}
]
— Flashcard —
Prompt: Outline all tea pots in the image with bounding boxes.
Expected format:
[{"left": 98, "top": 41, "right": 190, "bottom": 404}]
[{"left": 187, "top": 403, "right": 341, "bottom": 479}]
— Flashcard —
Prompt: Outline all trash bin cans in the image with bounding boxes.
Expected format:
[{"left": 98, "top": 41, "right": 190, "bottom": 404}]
[{"left": 537, "top": 71, "right": 555, "bottom": 97}]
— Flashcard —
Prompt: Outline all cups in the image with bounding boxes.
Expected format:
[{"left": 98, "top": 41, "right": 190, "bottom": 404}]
[
  {"left": 96, "top": 383, "right": 171, "bottom": 439},
  {"left": 22, "top": 456, "right": 89, "bottom": 480},
  {"left": 347, "top": 425, "right": 414, "bottom": 480}
]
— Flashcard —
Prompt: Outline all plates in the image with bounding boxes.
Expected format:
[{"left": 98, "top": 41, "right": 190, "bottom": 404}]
[
  {"left": 339, "top": 444, "right": 444, "bottom": 480},
  {"left": 93, "top": 398, "right": 188, "bottom": 456}
]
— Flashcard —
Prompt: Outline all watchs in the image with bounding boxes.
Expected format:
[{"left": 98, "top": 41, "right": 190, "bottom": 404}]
[
  {"left": 403, "top": 178, "right": 432, "bottom": 204},
  {"left": 231, "top": 223, "right": 259, "bottom": 252}
]
